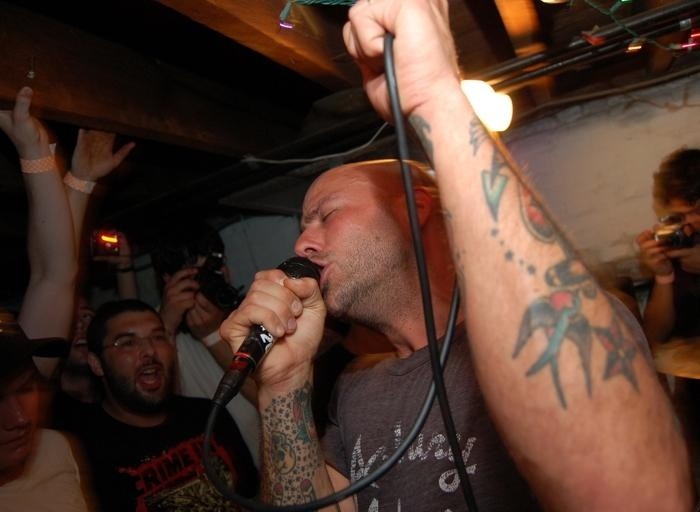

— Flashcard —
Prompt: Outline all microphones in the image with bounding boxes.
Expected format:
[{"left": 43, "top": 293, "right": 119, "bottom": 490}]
[{"left": 212, "top": 256, "right": 321, "bottom": 409}]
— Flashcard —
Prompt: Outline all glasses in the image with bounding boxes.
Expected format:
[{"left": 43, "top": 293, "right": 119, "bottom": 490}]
[{"left": 97, "top": 328, "right": 178, "bottom": 355}]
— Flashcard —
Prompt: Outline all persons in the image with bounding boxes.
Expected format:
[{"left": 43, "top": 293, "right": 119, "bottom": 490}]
[
  {"left": 218, "top": 2, "right": 700, "bottom": 512},
  {"left": 1, "top": 86, "right": 257, "bottom": 510},
  {"left": 644, "top": 148, "right": 700, "bottom": 340}
]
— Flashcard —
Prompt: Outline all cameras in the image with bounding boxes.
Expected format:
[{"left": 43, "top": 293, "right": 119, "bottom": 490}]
[
  {"left": 193, "top": 243, "right": 239, "bottom": 309},
  {"left": 654, "top": 223, "right": 700, "bottom": 252}
]
